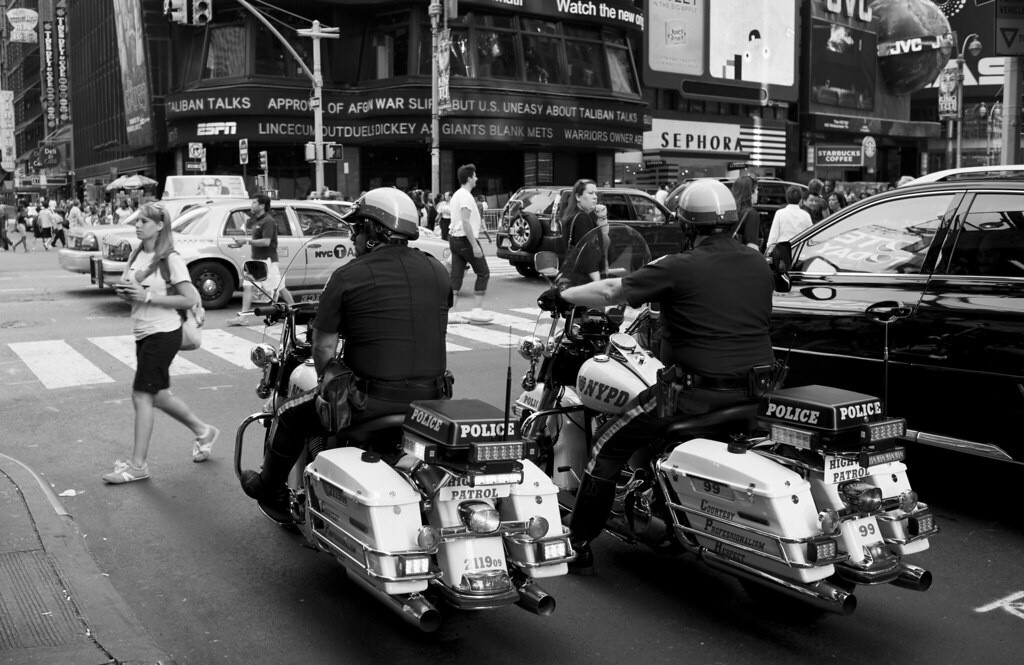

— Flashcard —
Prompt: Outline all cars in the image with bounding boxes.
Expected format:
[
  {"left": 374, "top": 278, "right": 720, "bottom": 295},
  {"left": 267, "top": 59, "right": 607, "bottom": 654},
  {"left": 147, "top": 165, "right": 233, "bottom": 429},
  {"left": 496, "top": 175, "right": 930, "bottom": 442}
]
[
  {"left": 764, "top": 165, "right": 1024, "bottom": 480},
  {"left": 58, "top": 175, "right": 250, "bottom": 274},
  {"left": 24, "top": 207, "right": 46, "bottom": 231},
  {"left": 89, "top": 198, "right": 356, "bottom": 309},
  {"left": 665, "top": 177, "right": 829, "bottom": 254},
  {"left": 308, "top": 200, "right": 438, "bottom": 240}
]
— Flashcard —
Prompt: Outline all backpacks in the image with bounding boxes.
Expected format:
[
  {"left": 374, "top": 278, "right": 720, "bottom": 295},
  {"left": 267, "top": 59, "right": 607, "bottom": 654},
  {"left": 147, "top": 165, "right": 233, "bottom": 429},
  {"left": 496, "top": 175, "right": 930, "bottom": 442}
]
[{"left": 160, "top": 250, "right": 205, "bottom": 351}]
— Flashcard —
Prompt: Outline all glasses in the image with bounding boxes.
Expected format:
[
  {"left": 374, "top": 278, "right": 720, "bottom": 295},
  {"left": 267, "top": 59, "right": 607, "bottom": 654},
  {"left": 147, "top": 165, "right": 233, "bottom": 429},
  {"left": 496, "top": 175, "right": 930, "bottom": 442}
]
[
  {"left": 351, "top": 224, "right": 365, "bottom": 235},
  {"left": 147, "top": 201, "right": 164, "bottom": 220}
]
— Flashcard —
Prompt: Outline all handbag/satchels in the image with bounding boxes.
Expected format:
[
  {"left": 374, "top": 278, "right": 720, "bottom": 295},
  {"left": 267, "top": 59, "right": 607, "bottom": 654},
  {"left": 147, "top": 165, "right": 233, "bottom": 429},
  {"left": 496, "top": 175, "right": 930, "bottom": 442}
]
[
  {"left": 557, "top": 261, "right": 591, "bottom": 286},
  {"left": 316, "top": 358, "right": 353, "bottom": 431}
]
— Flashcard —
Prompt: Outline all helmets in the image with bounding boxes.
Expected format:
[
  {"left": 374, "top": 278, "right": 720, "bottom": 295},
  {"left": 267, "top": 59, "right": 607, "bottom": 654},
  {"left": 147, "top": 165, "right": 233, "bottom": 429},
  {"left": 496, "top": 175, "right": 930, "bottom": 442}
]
[
  {"left": 676, "top": 179, "right": 740, "bottom": 224},
  {"left": 340, "top": 187, "right": 419, "bottom": 240}
]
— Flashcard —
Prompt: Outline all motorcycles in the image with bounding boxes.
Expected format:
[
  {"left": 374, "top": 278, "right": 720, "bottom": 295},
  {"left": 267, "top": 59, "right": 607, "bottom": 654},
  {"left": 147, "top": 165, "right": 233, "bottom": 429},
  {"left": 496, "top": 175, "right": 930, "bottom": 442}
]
[
  {"left": 233, "top": 231, "right": 576, "bottom": 633},
  {"left": 510, "top": 204, "right": 938, "bottom": 616}
]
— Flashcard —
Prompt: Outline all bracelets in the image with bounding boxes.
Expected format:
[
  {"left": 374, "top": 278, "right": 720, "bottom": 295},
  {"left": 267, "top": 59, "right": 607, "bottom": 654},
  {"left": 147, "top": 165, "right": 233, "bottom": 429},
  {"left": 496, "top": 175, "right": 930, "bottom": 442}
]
[{"left": 145, "top": 291, "right": 152, "bottom": 304}]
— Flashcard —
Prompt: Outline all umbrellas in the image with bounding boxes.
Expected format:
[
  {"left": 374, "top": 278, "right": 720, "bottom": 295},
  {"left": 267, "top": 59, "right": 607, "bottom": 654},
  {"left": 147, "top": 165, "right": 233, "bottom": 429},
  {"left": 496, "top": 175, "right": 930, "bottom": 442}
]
[{"left": 106, "top": 172, "right": 159, "bottom": 192}]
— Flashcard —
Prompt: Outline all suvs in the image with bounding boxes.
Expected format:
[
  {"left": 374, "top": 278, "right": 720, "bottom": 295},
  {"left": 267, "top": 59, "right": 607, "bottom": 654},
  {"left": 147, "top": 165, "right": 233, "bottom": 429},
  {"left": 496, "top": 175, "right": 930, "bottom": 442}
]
[{"left": 495, "top": 186, "right": 688, "bottom": 281}]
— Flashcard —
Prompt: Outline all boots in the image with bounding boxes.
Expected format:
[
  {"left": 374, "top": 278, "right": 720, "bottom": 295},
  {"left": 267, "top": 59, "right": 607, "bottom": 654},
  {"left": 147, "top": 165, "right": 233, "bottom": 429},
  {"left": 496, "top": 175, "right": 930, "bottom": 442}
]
[
  {"left": 240, "top": 440, "right": 292, "bottom": 506},
  {"left": 570, "top": 469, "right": 615, "bottom": 575}
]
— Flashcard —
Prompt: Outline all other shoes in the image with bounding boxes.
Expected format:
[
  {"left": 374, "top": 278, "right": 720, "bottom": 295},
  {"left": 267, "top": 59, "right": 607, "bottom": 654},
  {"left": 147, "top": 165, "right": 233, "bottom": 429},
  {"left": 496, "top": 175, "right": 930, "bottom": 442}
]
[
  {"left": 227, "top": 312, "right": 249, "bottom": 326},
  {"left": 471, "top": 308, "right": 494, "bottom": 322},
  {"left": 450, "top": 314, "right": 469, "bottom": 325},
  {"left": 1, "top": 242, "right": 69, "bottom": 253}
]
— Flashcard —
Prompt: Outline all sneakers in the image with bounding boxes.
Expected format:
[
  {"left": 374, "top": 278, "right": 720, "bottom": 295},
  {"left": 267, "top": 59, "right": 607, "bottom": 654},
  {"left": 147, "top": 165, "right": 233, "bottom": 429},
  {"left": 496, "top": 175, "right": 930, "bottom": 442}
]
[
  {"left": 103, "top": 459, "right": 149, "bottom": 483},
  {"left": 193, "top": 424, "right": 219, "bottom": 461}
]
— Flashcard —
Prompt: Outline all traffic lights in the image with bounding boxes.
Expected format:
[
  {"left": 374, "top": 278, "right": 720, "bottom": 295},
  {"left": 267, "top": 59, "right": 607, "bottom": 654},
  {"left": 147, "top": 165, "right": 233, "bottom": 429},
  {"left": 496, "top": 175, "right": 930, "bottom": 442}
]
[
  {"left": 188, "top": 0, "right": 213, "bottom": 25},
  {"left": 168, "top": 0, "right": 187, "bottom": 24}
]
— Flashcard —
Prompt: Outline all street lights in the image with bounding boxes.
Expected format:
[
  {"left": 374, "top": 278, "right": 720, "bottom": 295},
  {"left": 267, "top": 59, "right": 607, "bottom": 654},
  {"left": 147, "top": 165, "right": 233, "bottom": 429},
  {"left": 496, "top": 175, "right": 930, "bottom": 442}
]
[{"left": 940, "top": 32, "right": 982, "bottom": 180}]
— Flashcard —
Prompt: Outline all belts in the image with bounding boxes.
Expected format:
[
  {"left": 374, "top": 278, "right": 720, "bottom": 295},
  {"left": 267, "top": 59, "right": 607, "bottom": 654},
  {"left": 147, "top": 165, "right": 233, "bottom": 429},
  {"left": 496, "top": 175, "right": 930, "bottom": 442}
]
[
  {"left": 355, "top": 380, "right": 445, "bottom": 401},
  {"left": 692, "top": 372, "right": 749, "bottom": 390}
]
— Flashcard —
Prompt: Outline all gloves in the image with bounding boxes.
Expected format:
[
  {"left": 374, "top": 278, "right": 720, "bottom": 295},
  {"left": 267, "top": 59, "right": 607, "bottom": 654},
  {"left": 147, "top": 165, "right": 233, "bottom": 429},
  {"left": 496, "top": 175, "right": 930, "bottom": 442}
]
[{"left": 537, "top": 286, "right": 568, "bottom": 312}]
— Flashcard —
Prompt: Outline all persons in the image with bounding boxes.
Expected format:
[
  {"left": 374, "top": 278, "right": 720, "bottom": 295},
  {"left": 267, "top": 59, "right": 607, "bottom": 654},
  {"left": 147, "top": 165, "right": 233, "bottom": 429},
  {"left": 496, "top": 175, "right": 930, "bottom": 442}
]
[
  {"left": 303, "top": 186, "right": 494, "bottom": 244},
  {"left": 557, "top": 175, "right": 914, "bottom": 315},
  {"left": 0, "top": 197, "right": 142, "bottom": 253},
  {"left": 447, "top": 163, "right": 494, "bottom": 324},
  {"left": 240, "top": 188, "right": 461, "bottom": 513},
  {"left": 196, "top": 178, "right": 231, "bottom": 195},
  {"left": 100, "top": 203, "right": 218, "bottom": 483},
  {"left": 537, "top": 176, "right": 777, "bottom": 577},
  {"left": 227, "top": 194, "right": 296, "bottom": 326}
]
